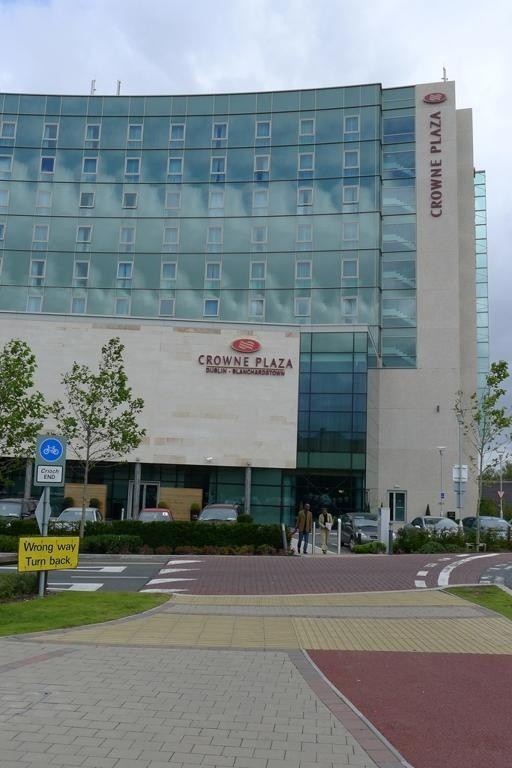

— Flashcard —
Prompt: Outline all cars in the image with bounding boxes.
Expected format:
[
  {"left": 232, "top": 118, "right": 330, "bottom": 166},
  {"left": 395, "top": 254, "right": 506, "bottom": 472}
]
[
  {"left": 336, "top": 509, "right": 397, "bottom": 551},
  {"left": 455, "top": 514, "right": 512, "bottom": 539},
  {"left": 52, "top": 505, "right": 105, "bottom": 532},
  {"left": 136, "top": 506, "right": 174, "bottom": 522},
  {"left": 402, "top": 515, "right": 459, "bottom": 536}
]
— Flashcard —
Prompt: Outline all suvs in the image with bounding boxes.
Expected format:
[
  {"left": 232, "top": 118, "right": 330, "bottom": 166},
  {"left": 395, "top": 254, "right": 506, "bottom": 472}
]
[{"left": 0, "top": 496, "right": 40, "bottom": 519}]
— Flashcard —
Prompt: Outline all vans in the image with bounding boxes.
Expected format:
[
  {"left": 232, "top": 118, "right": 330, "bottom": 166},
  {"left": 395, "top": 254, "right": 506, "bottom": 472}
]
[{"left": 194, "top": 500, "right": 241, "bottom": 521}]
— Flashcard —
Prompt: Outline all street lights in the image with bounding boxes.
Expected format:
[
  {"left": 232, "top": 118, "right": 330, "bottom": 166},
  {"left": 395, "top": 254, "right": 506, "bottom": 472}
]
[{"left": 438, "top": 445, "right": 448, "bottom": 516}]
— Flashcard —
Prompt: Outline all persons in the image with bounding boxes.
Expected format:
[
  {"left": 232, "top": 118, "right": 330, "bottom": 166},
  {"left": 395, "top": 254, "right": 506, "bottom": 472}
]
[
  {"left": 296, "top": 504, "right": 312, "bottom": 553},
  {"left": 319, "top": 508, "right": 333, "bottom": 554}
]
